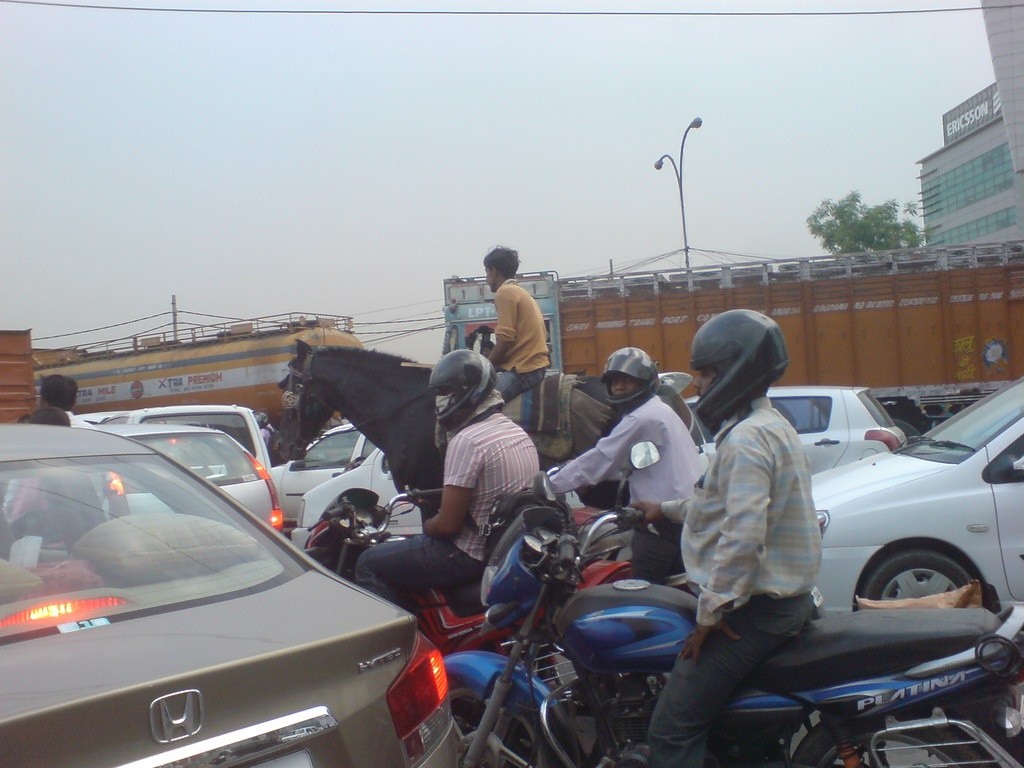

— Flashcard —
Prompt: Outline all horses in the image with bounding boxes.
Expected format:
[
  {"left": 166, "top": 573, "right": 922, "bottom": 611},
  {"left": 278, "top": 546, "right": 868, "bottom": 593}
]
[{"left": 261, "top": 336, "right": 713, "bottom": 586}]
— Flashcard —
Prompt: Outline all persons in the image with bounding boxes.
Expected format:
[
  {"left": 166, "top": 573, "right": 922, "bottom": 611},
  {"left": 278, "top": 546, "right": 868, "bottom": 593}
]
[
  {"left": 354, "top": 349, "right": 539, "bottom": 604},
  {"left": 7, "top": 372, "right": 102, "bottom": 545},
  {"left": 626, "top": 306, "right": 824, "bottom": 767},
  {"left": 254, "top": 411, "right": 280, "bottom": 456},
  {"left": 483, "top": 245, "right": 550, "bottom": 400},
  {"left": 489, "top": 346, "right": 704, "bottom": 587}
]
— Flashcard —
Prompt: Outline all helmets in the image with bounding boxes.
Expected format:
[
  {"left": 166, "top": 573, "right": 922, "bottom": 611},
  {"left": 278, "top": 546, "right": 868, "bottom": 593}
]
[
  {"left": 599, "top": 347, "right": 659, "bottom": 414},
  {"left": 689, "top": 309, "right": 789, "bottom": 431},
  {"left": 427, "top": 349, "right": 498, "bottom": 430}
]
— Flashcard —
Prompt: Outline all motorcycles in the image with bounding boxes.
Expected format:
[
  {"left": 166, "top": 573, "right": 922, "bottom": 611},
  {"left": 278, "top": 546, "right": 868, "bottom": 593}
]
[
  {"left": 445, "top": 509, "right": 1024, "bottom": 768},
  {"left": 304, "top": 488, "right": 828, "bottom": 768}
]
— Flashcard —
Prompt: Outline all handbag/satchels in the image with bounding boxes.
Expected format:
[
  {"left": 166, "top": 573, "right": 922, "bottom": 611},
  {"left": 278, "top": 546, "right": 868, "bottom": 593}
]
[{"left": 484, "top": 487, "right": 569, "bottom": 565}]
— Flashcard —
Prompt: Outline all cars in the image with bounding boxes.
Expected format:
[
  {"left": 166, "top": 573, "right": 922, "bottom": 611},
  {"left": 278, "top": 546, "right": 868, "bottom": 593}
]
[
  {"left": 59, "top": 401, "right": 441, "bottom": 539},
  {"left": 810, "top": 376, "right": 1024, "bottom": 617},
  {"left": 0, "top": 425, "right": 465, "bottom": 768},
  {"left": 674, "top": 386, "right": 902, "bottom": 475}
]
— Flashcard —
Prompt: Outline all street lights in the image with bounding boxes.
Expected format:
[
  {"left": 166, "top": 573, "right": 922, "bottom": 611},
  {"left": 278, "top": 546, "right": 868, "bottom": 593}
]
[{"left": 654, "top": 117, "right": 705, "bottom": 267}]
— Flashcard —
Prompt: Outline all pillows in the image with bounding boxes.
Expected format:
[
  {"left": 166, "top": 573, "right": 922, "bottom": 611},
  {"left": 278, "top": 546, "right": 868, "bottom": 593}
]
[{"left": 73, "top": 512, "right": 261, "bottom": 586}]
